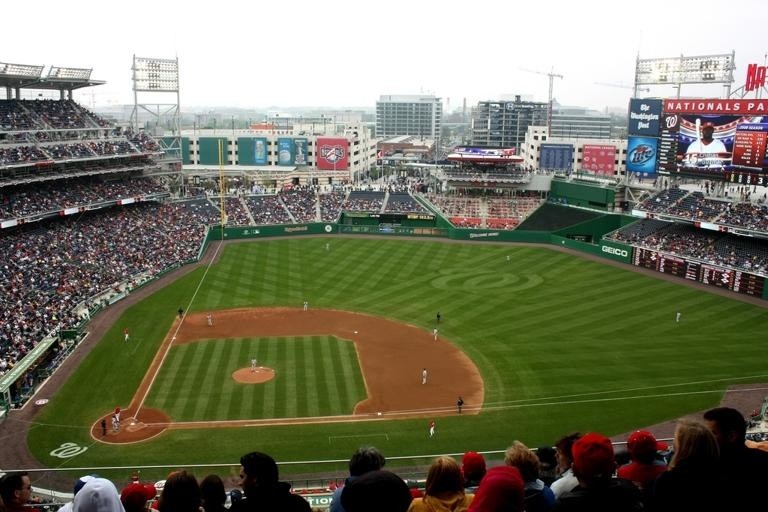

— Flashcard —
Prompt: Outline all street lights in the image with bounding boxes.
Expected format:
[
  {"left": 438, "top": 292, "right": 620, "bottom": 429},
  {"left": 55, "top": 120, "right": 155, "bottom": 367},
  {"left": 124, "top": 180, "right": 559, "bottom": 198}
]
[
  {"left": 632, "top": 49, "right": 736, "bottom": 103},
  {"left": 131, "top": 56, "right": 183, "bottom": 179}
]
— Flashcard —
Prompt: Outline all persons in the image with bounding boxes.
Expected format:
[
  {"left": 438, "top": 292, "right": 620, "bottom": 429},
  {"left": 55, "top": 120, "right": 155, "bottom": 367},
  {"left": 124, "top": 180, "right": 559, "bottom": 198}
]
[{"left": 1, "top": 100, "right": 768, "bottom": 512}]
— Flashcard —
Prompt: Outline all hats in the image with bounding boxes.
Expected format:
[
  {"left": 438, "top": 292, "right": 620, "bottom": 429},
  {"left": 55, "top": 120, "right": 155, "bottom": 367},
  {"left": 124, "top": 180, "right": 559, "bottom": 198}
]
[
  {"left": 74, "top": 474, "right": 157, "bottom": 510},
  {"left": 703, "top": 122, "right": 713, "bottom": 130},
  {"left": 463, "top": 452, "right": 486, "bottom": 475},
  {"left": 467, "top": 466, "right": 525, "bottom": 512},
  {"left": 571, "top": 431, "right": 669, "bottom": 474}
]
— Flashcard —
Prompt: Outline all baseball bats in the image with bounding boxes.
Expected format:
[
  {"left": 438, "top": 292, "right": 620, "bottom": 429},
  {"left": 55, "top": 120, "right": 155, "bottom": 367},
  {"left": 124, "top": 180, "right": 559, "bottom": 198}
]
[{"left": 694, "top": 118, "right": 702, "bottom": 153}]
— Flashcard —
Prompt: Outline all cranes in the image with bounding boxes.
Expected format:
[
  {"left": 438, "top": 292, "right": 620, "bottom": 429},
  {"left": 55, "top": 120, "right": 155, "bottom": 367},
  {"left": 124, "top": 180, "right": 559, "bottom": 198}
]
[{"left": 535, "top": 67, "right": 563, "bottom": 139}]
